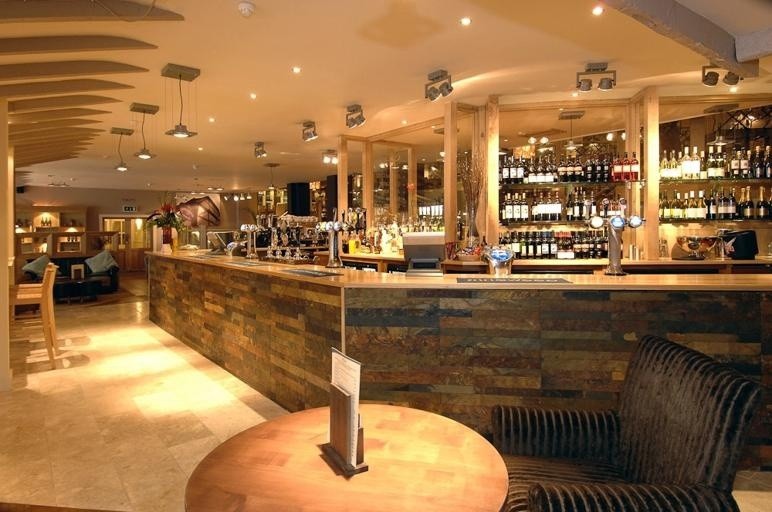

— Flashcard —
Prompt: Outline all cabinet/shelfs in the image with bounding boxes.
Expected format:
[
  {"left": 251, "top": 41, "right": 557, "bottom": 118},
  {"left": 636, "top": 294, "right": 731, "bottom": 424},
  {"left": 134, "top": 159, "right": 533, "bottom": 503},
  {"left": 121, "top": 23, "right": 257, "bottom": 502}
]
[
  {"left": 16, "top": 205, "right": 87, "bottom": 253},
  {"left": 254, "top": 102, "right": 772, "bottom": 274}
]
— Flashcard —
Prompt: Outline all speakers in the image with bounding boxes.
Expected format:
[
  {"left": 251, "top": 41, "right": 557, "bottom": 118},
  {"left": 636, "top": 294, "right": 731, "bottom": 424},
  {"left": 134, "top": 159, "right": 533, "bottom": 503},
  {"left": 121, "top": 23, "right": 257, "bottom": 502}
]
[
  {"left": 287, "top": 183, "right": 310, "bottom": 216},
  {"left": 327, "top": 175, "right": 352, "bottom": 208},
  {"left": 17, "top": 186, "right": 24, "bottom": 193}
]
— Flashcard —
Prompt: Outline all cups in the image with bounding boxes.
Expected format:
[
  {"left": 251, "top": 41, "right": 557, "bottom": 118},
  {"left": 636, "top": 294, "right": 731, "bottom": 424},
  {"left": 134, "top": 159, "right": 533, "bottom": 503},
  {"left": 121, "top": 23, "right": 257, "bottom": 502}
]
[
  {"left": 660, "top": 140, "right": 770, "bottom": 224},
  {"left": 486, "top": 251, "right": 518, "bottom": 277},
  {"left": 657, "top": 240, "right": 671, "bottom": 259}
]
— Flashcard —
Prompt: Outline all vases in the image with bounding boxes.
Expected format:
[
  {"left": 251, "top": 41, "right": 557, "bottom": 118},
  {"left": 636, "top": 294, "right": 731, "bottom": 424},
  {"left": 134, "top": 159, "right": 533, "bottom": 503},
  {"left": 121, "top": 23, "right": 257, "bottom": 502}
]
[{"left": 162, "top": 224, "right": 172, "bottom": 250}]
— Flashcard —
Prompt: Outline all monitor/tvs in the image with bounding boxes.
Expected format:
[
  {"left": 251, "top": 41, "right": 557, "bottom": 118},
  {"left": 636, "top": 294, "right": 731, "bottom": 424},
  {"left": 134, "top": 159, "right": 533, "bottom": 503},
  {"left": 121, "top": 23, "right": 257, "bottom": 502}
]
[
  {"left": 723, "top": 230, "right": 758, "bottom": 259},
  {"left": 402, "top": 232, "right": 445, "bottom": 276},
  {"left": 206, "top": 232, "right": 226, "bottom": 254}
]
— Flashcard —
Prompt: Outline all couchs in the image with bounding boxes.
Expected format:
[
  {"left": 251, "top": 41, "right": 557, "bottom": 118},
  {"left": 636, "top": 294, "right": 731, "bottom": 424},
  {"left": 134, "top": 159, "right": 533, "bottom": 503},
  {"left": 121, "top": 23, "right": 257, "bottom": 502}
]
[{"left": 24, "top": 257, "right": 119, "bottom": 293}]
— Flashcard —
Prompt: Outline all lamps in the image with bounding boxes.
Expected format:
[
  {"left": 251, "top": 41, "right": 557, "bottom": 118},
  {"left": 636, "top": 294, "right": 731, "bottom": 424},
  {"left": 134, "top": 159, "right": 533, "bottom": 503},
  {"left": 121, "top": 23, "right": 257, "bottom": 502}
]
[
  {"left": 575, "top": 62, "right": 617, "bottom": 91},
  {"left": 223, "top": 191, "right": 253, "bottom": 201},
  {"left": 110, "top": 62, "right": 201, "bottom": 172},
  {"left": 702, "top": 62, "right": 739, "bottom": 87},
  {"left": 425, "top": 70, "right": 453, "bottom": 100}
]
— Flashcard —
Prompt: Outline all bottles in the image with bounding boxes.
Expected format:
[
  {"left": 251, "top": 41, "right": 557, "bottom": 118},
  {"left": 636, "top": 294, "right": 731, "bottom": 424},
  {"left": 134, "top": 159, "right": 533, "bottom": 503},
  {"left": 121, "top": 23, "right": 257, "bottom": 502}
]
[
  {"left": 335, "top": 206, "right": 469, "bottom": 254},
  {"left": 493, "top": 152, "right": 643, "bottom": 261}
]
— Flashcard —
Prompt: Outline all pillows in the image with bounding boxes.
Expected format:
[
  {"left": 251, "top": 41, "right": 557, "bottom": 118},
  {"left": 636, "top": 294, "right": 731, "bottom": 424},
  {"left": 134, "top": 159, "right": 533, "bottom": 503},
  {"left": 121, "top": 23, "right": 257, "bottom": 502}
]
[
  {"left": 21, "top": 255, "right": 62, "bottom": 277},
  {"left": 85, "top": 250, "right": 119, "bottom": 272}
]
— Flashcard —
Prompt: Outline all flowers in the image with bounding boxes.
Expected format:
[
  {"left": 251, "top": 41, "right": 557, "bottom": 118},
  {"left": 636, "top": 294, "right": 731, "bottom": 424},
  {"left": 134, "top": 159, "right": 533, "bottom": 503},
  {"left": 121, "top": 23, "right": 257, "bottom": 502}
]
[{"left": 141, "top": 192, "right": 188, "bottom": 234}]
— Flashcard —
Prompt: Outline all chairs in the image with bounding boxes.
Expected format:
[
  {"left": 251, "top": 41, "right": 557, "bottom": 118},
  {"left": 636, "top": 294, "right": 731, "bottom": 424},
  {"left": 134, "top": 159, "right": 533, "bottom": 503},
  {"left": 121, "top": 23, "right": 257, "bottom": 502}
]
[
  {"left": 492, "top": 334, "right": 768, "bottom": 512},
  {"left": 9, "top": 262, "right": 59, "bottom": 370}
]
